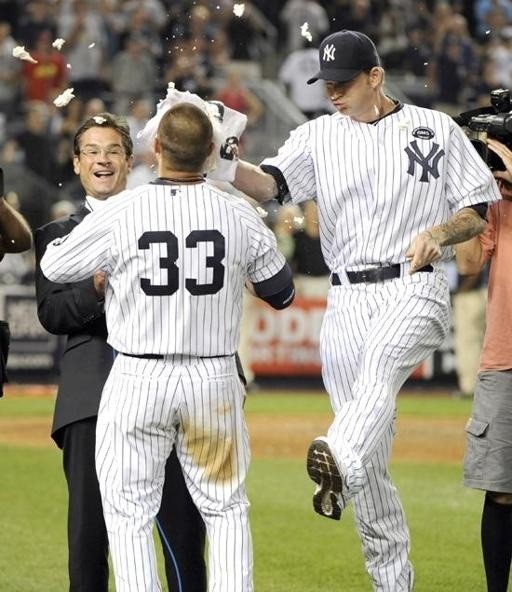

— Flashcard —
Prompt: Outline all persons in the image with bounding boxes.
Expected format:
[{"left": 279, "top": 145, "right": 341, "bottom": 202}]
[
  {"left": 40, "top": 104, "right": 299, "bottom": 591},
  {"left": 445, "top": 131, "right": 511, "bottom": 592},
  {"left": 33, "top": 108, "right": 207, "bottom": 591},
  {"left": 223, "top": 30, "right": 505, "bottom": 592},
  {"left": 2, "top": 168, "right": 34, "bottom": 399},
  {"left": 0, "top": 0, "right": 512, "bottom": 292}
]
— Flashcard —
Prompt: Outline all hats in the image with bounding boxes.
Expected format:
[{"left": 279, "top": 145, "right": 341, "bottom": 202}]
[{"left": 307, "top": 30, "right": 381, "bottom": 85}]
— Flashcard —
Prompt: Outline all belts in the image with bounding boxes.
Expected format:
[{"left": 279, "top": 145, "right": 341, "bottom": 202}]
[
  {"left": 123, "top": 353, "right": 229, "bottom": 360},
  {"left": 332, "top": 263, "right": 433, "bottom": 285}
]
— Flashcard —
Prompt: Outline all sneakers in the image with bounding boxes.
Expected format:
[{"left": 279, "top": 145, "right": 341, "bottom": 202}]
[{"left": 308, "top": 436, "right": 345, "bottom": 520}]
